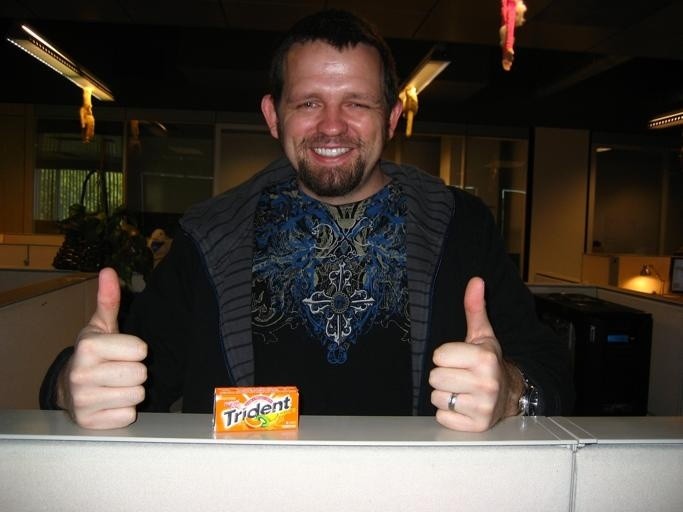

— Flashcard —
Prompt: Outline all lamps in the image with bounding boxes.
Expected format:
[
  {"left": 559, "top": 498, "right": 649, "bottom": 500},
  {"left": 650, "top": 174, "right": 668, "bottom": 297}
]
[
  {"left": 6, "top": 24, "right": 116, "bottom": 105},
  {"left": 637, "top": 262, "right": 665, "bottom": 296},
  {"left": 396, "top": 40, "right": 458, "bottom": 109}
]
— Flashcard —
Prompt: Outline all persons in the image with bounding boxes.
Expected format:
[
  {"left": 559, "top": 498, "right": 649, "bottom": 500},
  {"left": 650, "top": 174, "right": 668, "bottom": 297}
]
[{"left": 38, "top": 8, "right": 580, "bottom": 432}]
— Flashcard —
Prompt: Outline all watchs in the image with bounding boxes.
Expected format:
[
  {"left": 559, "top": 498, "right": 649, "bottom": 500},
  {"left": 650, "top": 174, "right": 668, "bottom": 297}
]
[{"left": 510, "top": 364, "right": 540, "bottom": 419}]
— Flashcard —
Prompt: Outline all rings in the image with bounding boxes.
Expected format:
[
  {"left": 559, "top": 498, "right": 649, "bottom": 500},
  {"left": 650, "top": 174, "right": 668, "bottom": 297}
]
[{"left": 447, "top": 390, "right": 459, "bottom": 412}]
